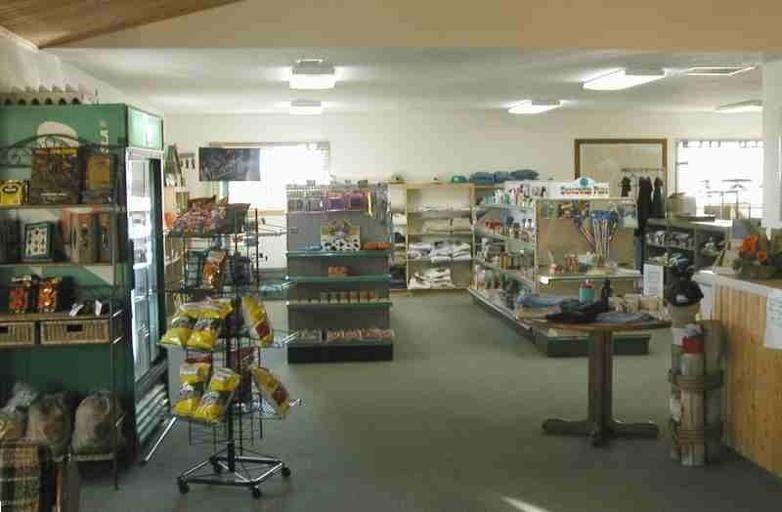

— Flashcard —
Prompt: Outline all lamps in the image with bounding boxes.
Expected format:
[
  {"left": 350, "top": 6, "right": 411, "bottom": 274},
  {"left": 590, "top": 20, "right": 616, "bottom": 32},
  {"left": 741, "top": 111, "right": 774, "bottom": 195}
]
[
  {"left": 288, "top": 58, "right": 339, "bottom": 92},
  {"left": 508, "top": 97, "right": 561, "bottom": 116},
  {"left": 580, "top": 66, "right": 666, "bottom": 92}
]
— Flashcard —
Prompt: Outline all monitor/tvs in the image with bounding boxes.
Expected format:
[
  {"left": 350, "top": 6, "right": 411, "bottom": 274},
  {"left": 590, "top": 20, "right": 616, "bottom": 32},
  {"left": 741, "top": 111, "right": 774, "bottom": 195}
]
[{"left": 197, "top": 147, "right": 261, "bottom": 182}]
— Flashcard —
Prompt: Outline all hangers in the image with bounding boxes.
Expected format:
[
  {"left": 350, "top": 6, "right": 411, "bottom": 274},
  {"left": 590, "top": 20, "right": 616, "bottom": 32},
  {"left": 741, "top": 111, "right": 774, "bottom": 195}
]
[{"left": 620, "top": 167, "right": 663, "bottom": 189}]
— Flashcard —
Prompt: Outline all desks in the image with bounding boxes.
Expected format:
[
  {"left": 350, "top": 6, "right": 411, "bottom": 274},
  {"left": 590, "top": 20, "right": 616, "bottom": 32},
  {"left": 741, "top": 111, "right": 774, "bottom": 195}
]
[{"left": 515, "top": 303, "right": 671, "bottom": 449}]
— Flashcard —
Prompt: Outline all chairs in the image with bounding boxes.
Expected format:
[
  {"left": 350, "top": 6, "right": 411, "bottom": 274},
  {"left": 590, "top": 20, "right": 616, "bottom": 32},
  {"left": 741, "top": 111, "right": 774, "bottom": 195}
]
[{"left": 3, "top": 442, "right": 81, "bottom": 512}]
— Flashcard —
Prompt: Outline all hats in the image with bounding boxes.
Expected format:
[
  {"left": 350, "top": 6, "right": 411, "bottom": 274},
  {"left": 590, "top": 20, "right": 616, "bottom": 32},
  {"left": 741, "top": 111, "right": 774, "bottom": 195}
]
[{"left": 666, "top": 279, "right": 704, "bottom": 306}]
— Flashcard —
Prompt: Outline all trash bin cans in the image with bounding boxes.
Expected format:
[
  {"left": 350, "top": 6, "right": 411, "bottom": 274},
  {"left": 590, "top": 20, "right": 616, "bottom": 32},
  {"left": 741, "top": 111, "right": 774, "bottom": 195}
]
[{"left": 667, "top": 281, "right": 704, "bottom": 328}]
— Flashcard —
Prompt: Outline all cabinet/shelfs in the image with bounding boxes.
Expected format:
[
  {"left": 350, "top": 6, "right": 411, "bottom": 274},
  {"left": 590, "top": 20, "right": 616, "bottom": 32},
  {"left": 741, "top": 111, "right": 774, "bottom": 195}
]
[
  {"left": 5, "top": 105, "right": 173, "bottom": 469},
  {"left": 467, "top": 191, "right": 651, "bottom": 359},
  {"left": 643, "top": 213, "right": 732, "bottom": 324},
  {"left": 402, "top": 181, "right": 476, "bottom": 294},
  {"left": 164, "top": 210, "right": 293, "bottom": 496},
  {"left": 283, "top": 183, "right": 395, "bottom": 364}
]
